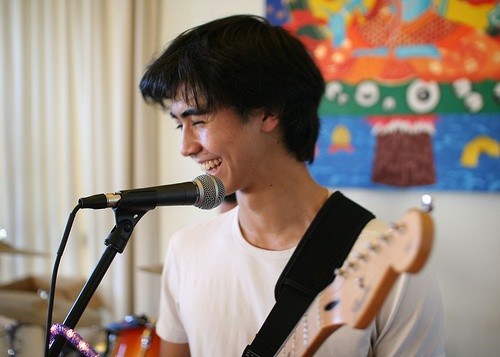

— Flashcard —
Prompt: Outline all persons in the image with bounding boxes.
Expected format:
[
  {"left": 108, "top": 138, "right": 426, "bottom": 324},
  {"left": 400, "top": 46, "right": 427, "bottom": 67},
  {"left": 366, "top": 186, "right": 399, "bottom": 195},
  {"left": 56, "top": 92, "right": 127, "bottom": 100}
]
[{"left": 138, "top": 13, "right": 445, "bottom": 357}]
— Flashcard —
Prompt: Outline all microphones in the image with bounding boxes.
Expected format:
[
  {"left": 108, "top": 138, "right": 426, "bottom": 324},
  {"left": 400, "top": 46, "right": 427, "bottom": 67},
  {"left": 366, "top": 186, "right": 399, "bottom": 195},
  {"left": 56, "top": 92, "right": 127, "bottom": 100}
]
[{"left": 80, "top": 174, "right": 225, "bottom": 210}]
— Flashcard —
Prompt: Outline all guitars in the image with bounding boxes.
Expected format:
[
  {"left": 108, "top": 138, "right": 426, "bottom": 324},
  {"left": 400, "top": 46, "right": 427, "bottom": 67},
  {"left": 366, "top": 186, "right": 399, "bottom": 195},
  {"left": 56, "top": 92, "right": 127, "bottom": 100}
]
[{"left": 272, "top": 194, "right": 434, "bottom": 357}]
